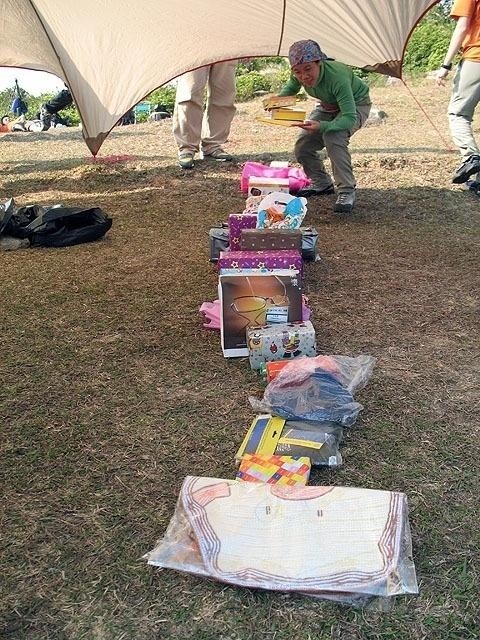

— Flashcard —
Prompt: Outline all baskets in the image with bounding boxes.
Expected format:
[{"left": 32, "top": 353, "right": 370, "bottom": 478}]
[{"left": 261, "top": 95, "right": 296, "bottom": 110}]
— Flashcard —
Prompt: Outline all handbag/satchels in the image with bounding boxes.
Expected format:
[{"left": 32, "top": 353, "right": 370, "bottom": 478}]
[{"left": 219, "top": 275, "right": 303, "bottom": 358}]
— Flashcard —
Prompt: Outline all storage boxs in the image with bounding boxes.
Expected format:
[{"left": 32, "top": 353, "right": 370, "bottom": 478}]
[{"left": 218, "top": 176, "right": 315, "bottom": 370}]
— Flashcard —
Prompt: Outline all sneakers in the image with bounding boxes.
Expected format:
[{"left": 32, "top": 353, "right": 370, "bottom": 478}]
[
  {"left": 296, "top": 183, "right": 334, "bottom": 198},
  {"left": 178, "top": 148, "right": 234, "bottom": 169},
  {"left": 452, "top": 157, "right": 480, "bottom": 197},
  {"left": 333, "top": 191, "right": 356, "bottom": 211}
]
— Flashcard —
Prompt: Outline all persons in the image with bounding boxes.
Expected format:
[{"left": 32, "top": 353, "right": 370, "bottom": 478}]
[
  {"left": 0, "top": 115, "right": 44, "bottom": 133},
  {"left": 170, "top": 59, "right": 239, "bottom": 169},
  {"left": 37, "top": 82, "right": 74, "bottom": 132},
  {"left": 270, "top": 38, "right": 373, "bottom": 214},
  {"left": 436, "top": 0, "right": 480, "bottom": 198}
]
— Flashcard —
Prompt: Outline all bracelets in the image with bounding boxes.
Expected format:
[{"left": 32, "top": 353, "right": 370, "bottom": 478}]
[{"left": 439, "top": 63, "right": 452, "bottom": 71}]
